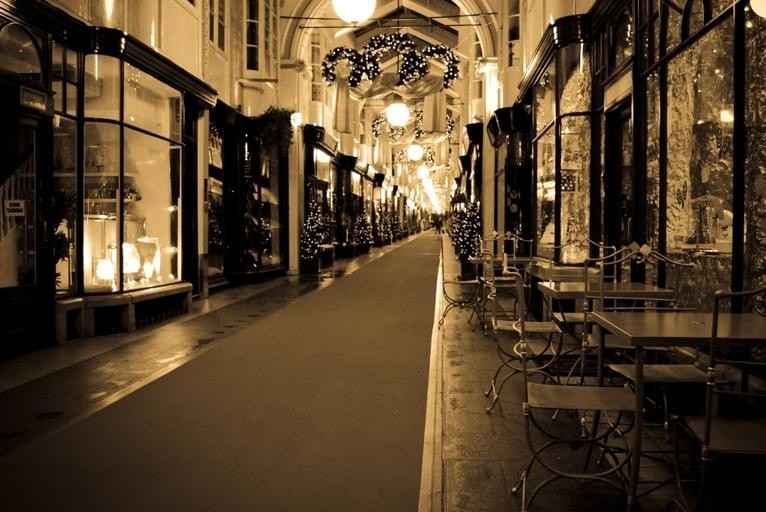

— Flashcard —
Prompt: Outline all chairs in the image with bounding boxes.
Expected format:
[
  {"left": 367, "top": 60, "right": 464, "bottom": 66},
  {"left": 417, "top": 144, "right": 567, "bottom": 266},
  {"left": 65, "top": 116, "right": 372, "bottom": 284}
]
[
  {"left": 435, "top": 230, "right": 534, "bottom": 336},
  {"left": 485, "top": 236, "right": 700, "bottom": 438},
  {"left": 511, "top": 244, "right": 765, "bottom": 512}
]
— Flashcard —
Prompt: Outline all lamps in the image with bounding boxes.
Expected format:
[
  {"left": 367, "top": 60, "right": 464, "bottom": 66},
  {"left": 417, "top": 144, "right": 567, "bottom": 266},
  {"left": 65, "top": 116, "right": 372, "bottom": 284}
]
[
  {"left": 333, "top": 1, "right": 376, "bottom": 28},
  {"left": 165, "top": 204, "right": 178, "bottom": 256},
  {"left": 110, "top": 273, "right": 176, "bottom": 291}
]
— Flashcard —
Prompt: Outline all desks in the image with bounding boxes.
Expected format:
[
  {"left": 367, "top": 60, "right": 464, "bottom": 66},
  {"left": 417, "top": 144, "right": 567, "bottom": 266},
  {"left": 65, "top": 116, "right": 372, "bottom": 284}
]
[
  {"left": 582, "top": 308, "right": 764, "bottom": 509},
  {"left": 466, "top": 255, "right": 534, "bottom": 335},
  {"left": 536, "top": 279, "right": 677, "bottom": 418}
]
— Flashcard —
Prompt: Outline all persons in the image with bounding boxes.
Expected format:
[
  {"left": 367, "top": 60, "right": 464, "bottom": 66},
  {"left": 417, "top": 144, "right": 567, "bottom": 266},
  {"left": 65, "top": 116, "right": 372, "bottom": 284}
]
[
  {"left": 434, "top": 217, "right": 442, "bottom": 235},
  {"left": 687, "top": 120, "right": 732, "bottom": 237}
]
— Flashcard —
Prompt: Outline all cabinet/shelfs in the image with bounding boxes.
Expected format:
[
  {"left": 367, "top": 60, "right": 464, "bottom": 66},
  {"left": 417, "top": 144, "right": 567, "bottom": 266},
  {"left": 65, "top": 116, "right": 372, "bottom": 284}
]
[{"left": 52, "top": 171, "right": 134, "bottom": 203}]
